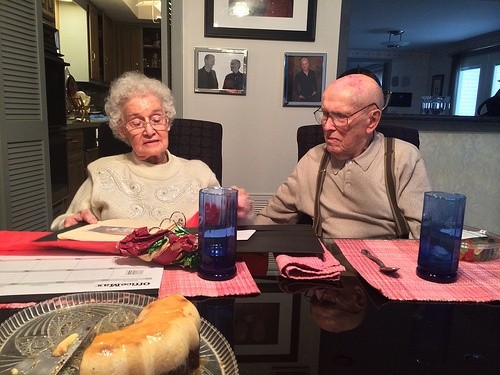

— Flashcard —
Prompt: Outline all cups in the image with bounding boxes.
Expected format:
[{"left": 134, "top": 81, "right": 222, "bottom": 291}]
[
  {"left": 414, "top": 191, "right": 466, "bottom": 285},
  {"left": 197, "top": 187, "right": 238, "bottom": 281}
]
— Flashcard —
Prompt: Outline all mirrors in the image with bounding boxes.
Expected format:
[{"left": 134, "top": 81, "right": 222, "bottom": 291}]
[{"left": 336, "top": 0, "right": 500, "bottom": 133}]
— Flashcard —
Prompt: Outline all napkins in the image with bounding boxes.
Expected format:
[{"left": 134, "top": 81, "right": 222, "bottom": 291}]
[{"left": 237, "top": 230, "right": 256, "bottom": 240}]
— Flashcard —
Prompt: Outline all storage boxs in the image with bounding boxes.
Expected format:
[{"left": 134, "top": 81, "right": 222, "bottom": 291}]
[{"left": 459, "top": 224, "right": 500, "bottom": 263}]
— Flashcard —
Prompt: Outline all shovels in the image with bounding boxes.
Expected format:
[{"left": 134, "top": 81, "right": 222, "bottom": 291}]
[{"left": 8, "top": 313, "right": 104, "bottom": 375}]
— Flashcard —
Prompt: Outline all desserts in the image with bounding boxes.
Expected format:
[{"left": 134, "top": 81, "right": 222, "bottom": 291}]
[{"left": 79, "top": 294, "right": 201, "bottom": 375}]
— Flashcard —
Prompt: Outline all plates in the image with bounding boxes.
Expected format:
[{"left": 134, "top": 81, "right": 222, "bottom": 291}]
[{"left": 0, "top": 292, "right": 238, "bottom": 375}]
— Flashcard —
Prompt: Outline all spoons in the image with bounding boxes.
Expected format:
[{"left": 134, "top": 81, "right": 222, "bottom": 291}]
[{"left": 360, "top": 249, "right": 400, "bottom": 272}]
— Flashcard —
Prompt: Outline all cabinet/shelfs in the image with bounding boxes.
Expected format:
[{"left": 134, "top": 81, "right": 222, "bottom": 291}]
[
  {"left": 52, "top": 130, "right": 114, "bottom": 218},
  {"left": 59, "top": 4, "right": 161, "bottom": 91}
]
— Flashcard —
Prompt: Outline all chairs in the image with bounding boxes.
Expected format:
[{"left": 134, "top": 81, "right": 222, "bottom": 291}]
[
  {"left": 168, "top": 120, "right": 223, "bottom": 187},
  {"left": 297, "top": 124, "right": 419, "bottom": 225}
]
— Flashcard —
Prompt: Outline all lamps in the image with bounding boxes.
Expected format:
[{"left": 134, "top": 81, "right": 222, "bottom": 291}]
[{"left": 381, "top": 29, "right": 410, "bottom": 48}]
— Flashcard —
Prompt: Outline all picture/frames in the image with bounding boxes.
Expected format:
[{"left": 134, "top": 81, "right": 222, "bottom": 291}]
[
  {"left": 194, "top": 47, "right": 248, "bottom": 96},
  {"left": 283, "top": 52, "right": 327, "bottom": 107},
  {"left": 204, "top": 0, "right": 317, "bottom": 42}
]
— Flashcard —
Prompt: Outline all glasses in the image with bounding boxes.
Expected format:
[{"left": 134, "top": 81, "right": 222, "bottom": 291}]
[
  {"left": 123, "top": 117, "right": 170, "bottom": 135},
  {"left": 313, "top": 103, "right": 381, "bottom": 127},
  {"left": 303, "top": 288, "right": 365, "bottom": 314}
]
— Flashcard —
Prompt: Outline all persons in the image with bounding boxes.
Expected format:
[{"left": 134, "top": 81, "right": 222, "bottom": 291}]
[
  {"left": 294, "top": 58, "right": 317, "bottom": 102},
  {"left": 222, "top": 59, "right": 244, "bottom": 90},
  {"left": 48, "top": 71, "right": 253, "bottom": 231},
  {"left": 275, "top": 239, "right": 389, "bottom": 332},
  {"left": 198, "top": 54, "right": 219, "bottom": 89},
  {"left": 251, "top": 73, "right": 434, "bottom": 375}
]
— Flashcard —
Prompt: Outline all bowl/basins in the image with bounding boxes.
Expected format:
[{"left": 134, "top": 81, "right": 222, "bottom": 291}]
[{"left": 458, "top": 225, "right": 500, "bottom": 261}]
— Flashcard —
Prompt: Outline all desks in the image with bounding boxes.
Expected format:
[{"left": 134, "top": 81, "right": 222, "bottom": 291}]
[{"left": 0, "top": 230, "right": 500, "bottom": 375}]
